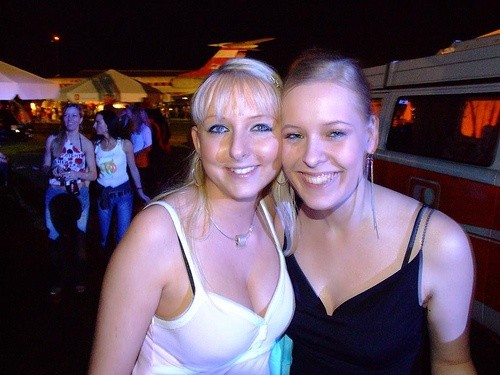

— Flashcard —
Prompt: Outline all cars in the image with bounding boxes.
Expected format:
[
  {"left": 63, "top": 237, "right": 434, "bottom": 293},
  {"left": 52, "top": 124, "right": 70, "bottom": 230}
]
[{"left": 0, "top": 100, "right": 35, "bottom": 142}]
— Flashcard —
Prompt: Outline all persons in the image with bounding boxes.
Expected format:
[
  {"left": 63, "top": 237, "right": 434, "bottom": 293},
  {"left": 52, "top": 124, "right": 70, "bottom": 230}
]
[
  {"left": 88, "top": 58, "right": 296, "bottom": 375},
  {"left": 280, "top": 50, "right": 478, "bottom": 375},
  {"left": 39, "top": 103, "right": 175, "bottom": 260}
]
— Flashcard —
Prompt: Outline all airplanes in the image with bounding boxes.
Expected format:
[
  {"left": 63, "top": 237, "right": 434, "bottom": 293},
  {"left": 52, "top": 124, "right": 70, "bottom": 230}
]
[{"left": 45, "top": 37, "right": 277, "bottom": 103}]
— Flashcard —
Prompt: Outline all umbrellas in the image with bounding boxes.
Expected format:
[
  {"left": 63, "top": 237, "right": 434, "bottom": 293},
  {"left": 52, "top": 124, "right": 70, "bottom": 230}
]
[
  {"left": 0, "top": 61, "right": 61, "bottom": 100},
  {"left": 60, "top": 68, "right": 173, "bottom": 104}
]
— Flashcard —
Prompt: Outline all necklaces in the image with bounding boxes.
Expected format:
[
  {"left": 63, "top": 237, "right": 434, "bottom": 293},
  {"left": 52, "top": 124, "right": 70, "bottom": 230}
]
[{"left": 203, "top": 203, "right": 254, "bottom": 247}]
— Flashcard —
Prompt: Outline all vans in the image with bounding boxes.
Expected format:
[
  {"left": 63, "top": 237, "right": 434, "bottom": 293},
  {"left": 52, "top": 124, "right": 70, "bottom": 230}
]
[{"left": 363, "top": 33, "right": 500, "bottom": 347}]
